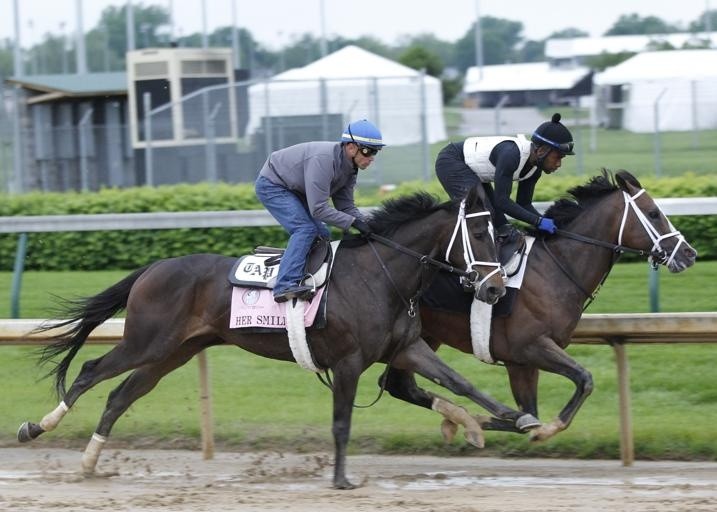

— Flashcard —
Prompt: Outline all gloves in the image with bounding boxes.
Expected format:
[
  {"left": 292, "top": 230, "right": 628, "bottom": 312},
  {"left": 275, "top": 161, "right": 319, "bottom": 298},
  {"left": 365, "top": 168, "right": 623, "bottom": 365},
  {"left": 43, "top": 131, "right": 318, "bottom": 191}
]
[
  {"left": 352, "top": 218, "right": 373, "bottom": 239},
  {"left": 537, "top": 217, "right": 557, "bottom": 234}
]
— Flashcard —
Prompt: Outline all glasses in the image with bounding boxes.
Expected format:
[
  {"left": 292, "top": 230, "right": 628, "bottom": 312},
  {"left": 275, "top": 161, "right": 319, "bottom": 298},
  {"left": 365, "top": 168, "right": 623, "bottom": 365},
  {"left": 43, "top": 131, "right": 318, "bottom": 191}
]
[
  {"left": 349, "top": 124, "right": 381, "bottom": 156},
  {"left": 532, "top": 132, "right": 574, "bottom": 152}
]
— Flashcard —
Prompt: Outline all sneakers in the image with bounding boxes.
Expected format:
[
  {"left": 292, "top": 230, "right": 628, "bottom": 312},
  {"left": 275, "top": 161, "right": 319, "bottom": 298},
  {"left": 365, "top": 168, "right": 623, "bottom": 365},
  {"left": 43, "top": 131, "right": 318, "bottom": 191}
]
[{"left": 274, "top": 285, "right": 313, "bottom": 303}]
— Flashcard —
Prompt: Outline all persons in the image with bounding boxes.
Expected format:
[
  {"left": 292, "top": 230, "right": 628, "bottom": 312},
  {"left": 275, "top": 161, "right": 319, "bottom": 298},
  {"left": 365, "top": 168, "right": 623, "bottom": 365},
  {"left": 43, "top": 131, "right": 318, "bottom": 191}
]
[
  {"left": 436, "top": 113, "right": 576, "bottom": 269},
  {"left": 253, "top": 117, "right": 387, "bottom": 304}
]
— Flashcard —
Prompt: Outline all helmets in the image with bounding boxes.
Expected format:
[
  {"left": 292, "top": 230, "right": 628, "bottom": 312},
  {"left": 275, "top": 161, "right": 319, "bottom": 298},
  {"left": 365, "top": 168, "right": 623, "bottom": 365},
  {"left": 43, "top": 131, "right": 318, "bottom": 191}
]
[
  {"left": 532, "top": 113, "right": 575, "bottom": 155},
  {"left": 341, "top": 119, "right": 386, "bottom": 146}
]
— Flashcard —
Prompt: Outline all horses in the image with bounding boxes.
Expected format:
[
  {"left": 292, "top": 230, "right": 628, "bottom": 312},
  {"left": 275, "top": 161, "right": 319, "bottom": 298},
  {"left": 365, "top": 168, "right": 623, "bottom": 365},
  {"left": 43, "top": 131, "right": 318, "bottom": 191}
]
[
  {"left": 16, "top": 181, "right": 545, "bottom": 491},
  {"left": 377, "top": 164, "right": 699, "bottom": 451}
]
